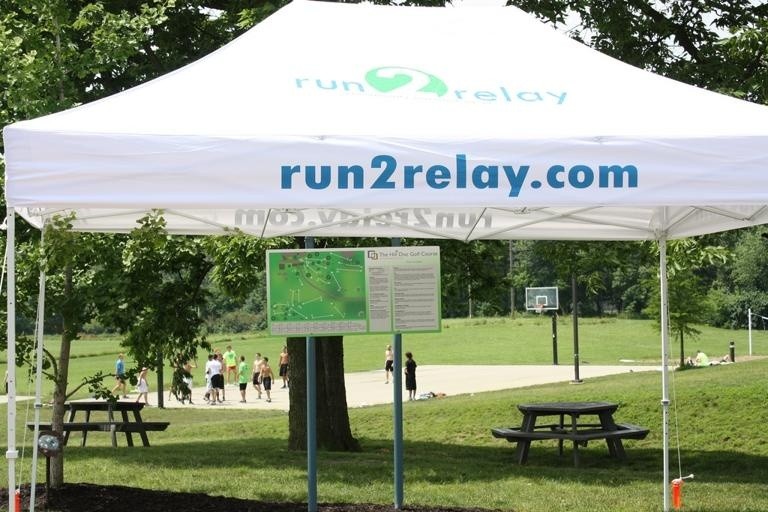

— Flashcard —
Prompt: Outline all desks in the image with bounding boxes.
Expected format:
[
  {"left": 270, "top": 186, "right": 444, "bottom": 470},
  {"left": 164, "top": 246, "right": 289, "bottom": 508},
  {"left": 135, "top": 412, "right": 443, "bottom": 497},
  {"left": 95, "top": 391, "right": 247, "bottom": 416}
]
[
  {"left": 62, "top": 401, "right": 149, "bottom": 447},
  {"left": 515, "top": 403, "right": 627, "bottom": 462}
]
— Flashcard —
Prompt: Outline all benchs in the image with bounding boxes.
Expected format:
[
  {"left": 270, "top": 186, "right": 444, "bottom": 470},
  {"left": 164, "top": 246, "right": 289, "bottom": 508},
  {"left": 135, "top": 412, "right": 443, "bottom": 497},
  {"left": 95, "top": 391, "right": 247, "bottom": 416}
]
[
  {"left": 27, "top": 422, "right": 171, "bottom": 449},
  {"left": 491, "top": 425, "right": 650, "bottom": 467}
]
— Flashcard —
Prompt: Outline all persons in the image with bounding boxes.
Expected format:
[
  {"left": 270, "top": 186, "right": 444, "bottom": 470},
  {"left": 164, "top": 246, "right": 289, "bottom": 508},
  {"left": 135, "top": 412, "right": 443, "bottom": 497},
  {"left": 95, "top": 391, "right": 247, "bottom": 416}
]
[
  {"left": 684, "top": 351, "right": 733, "bottom": 367},
  {"left": 383, "top": 344, "right": 394, "bottom": 384},
  {"left": 405, "top": 352, "right": 417, "bottom": 400},
  {"left": 110, "top": 345, "right": 288, "bottom": 406}
]
